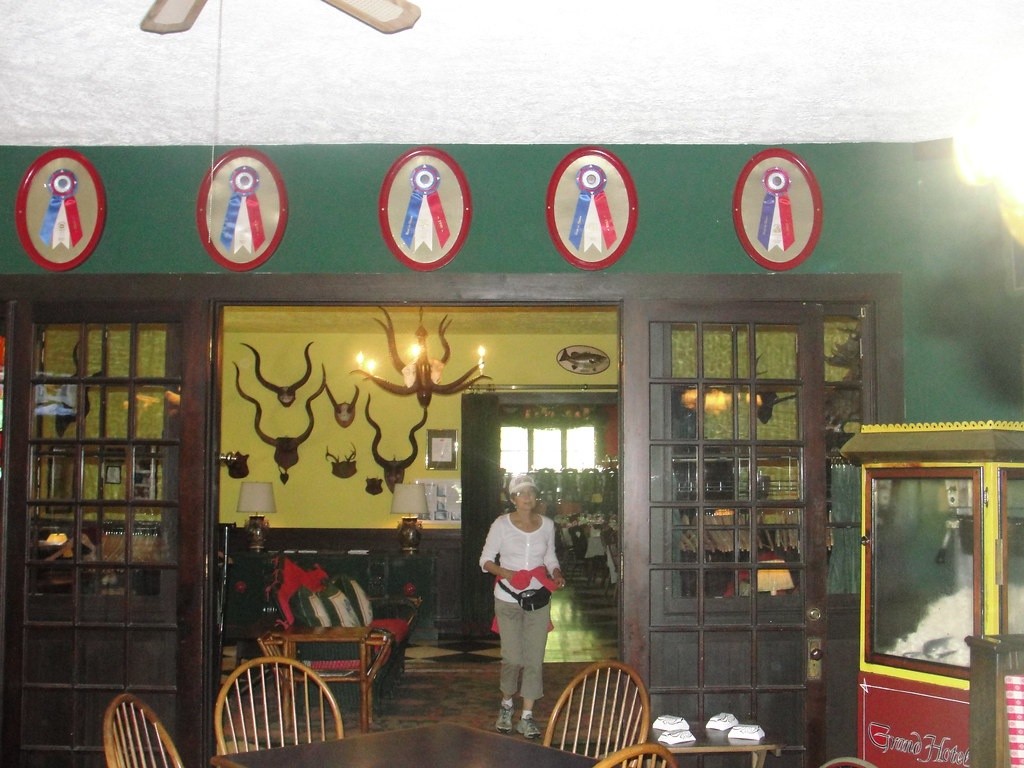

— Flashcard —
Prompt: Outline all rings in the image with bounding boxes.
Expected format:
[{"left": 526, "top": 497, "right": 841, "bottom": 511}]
[{"left": 562, "top": 585, "right": 565, "bottom": 587}]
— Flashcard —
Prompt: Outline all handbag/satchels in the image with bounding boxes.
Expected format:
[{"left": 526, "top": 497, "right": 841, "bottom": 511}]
[{"left": 498, "top": 580, "right": 552, "bottom": 611}]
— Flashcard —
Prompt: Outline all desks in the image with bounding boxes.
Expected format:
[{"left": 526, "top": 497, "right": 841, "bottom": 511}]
[
  {"left": 627, "top": 719, "right": 786, "bottom": 768},
  {"left": 210, "top": 722, "right": 600, "bottom": 768}
]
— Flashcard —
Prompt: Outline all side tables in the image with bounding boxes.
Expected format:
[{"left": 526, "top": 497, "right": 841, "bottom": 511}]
[{"left": 256, "top": 626, "right": 392, "bottom": 734}]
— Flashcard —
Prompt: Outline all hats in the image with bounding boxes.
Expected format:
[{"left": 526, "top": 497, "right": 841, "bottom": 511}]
[{"left": 509, "top": 474, "right": 540, "bottom": 495}]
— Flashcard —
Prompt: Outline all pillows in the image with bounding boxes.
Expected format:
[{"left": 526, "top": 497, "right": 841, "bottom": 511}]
[
  {"left": 317, "top": 583, "right": 361, "bottom": 627},
  {"left": 290, "top": 584, "right": 332, "bottom": 626},
  {"left": 329, "top": 576, "right": 374, "bottom": 626}
]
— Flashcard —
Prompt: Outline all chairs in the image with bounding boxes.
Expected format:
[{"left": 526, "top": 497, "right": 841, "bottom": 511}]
[
  {"left": 543, "top": 660, "right": 650, "bottom": 767},
  {"left": 103, "top": 693, "right": 185, "bottom": 768},
  {"left": 214, "top": 656, "right": 344, "bottom": 757}
]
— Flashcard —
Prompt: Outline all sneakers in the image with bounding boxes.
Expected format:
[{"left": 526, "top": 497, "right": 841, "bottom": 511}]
[
  {"left": 516, "top": 715, "right": 542, "bottom": 739},
  {"left": 495, "top": 705, "right": 515, "bottom": 734}
]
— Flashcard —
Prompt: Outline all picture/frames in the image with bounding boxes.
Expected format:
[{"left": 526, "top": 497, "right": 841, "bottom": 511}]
[{"left": 426, "top": 428, "right": 458, "bottom": 470}]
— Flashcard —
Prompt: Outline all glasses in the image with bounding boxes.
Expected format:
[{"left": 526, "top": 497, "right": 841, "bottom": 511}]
[{"left": 513, "top": 490, "right": 537, "bottom": 497}]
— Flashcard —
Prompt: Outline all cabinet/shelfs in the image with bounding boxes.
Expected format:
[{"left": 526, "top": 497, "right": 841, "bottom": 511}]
[{"left": 224, "top": 549, "right": 440, "bottom": 641}]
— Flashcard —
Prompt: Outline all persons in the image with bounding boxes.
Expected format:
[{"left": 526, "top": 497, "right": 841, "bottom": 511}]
[{"left": 479, "top": 475, "right": 566, "bottom": 739}]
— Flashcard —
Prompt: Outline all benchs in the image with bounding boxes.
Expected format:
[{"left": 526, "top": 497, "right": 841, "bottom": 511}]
[{"left": 264, "top": 549, "right": 418, "bottom": 717}]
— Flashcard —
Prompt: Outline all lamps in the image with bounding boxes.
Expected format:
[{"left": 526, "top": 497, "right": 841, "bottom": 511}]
[
  {"left": 350, "top": 307, "right": 493, "bottom": 406},
  {"left": 389, "top": 482, "right": 430, "bottom": 554},
  {"left": 236, "top": 482, "right": 276, "bottom": 549},
  {"left": 757, "top": 558, "right": 795, "bottom": 596}
]
